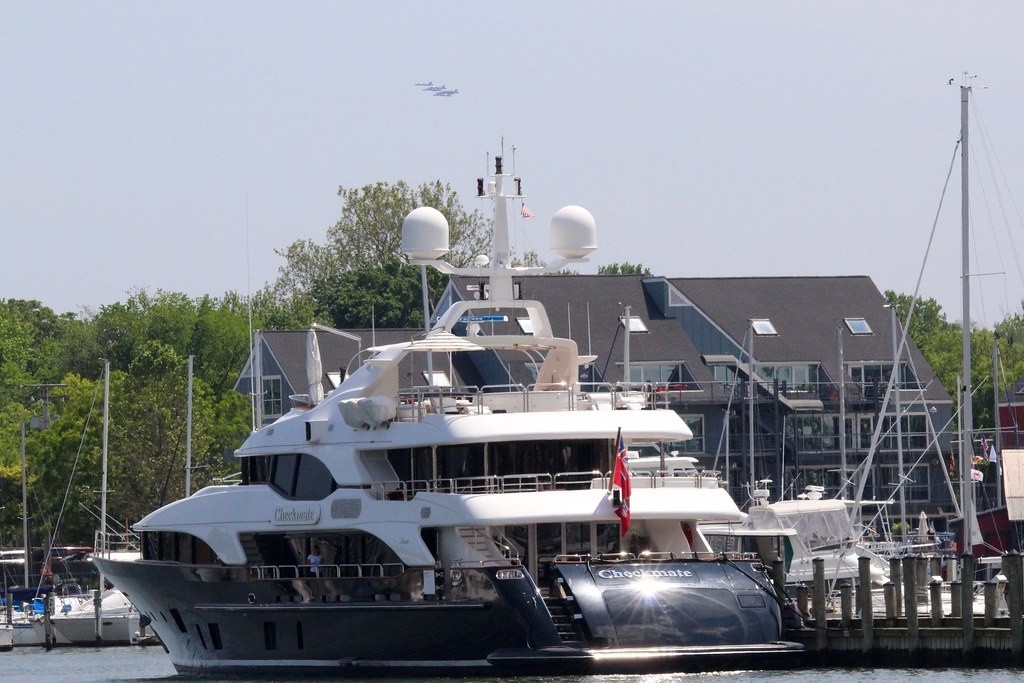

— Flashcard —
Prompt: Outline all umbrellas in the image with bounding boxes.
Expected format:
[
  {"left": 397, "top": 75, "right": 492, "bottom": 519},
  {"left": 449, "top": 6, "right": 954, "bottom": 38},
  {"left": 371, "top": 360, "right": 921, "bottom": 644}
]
[
  {"left": 305, "top": 329, "right": 325, "bottom": 407},
  {"left": 918, "top": 510, "right": 929, "bottom": 544}
]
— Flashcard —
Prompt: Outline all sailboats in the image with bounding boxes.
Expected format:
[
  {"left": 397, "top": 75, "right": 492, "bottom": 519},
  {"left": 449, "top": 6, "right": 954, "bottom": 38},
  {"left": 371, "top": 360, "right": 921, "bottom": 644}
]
[
  {"left": 0, "top": 355, "right": 209, "bottom": 650},
  {"left": 708, "top": 69, "right": 1023, "bottom": 577}
]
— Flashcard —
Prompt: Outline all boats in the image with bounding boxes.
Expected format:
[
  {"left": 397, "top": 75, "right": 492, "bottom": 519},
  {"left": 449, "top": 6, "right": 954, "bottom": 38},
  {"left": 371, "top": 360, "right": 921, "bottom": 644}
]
[{"left": 93, "top": 137, "right": 805, "bottom": 676}]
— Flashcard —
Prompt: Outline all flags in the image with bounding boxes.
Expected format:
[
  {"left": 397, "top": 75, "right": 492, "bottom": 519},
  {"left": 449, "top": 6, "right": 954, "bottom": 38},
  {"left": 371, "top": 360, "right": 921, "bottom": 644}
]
[
  {"left": 971, "top": 455, "right": 985, "bottom": 483},
  {"left": 949, "top": 451, "right": 956, "bottom": 481},
  {"left": 613, "top": 432, "right": 631, "bottom": 540},
  {"left": 982, "top": 433, "right": 997, "bottom": 463},
  {"left": 521, "top": 204, "right": 534, "bottom": 218}
]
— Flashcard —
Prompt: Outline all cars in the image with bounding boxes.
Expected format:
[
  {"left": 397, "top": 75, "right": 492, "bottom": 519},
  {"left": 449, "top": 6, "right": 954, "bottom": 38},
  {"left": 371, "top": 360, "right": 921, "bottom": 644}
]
[
  {"left": 51, "top": 548, "right": 93, "bottom": 563},
  {"left": 0, "top": 550, "right": 25, "bottom": 564},
  {"left": 905, "top": 531, "right": 956, "bottom": 550}
]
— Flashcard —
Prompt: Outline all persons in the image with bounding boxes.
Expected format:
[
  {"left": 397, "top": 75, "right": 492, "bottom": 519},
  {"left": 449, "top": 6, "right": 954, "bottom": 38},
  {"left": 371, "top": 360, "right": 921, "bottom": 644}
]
[{"left": 308, "top": 546, "right": 322, "bottom": 578}]
